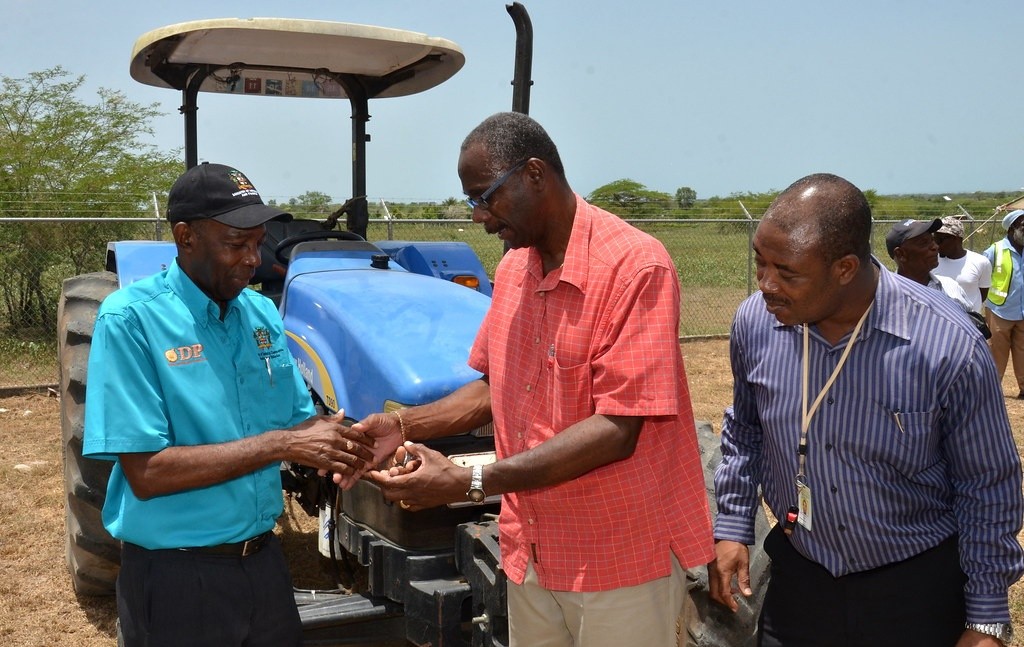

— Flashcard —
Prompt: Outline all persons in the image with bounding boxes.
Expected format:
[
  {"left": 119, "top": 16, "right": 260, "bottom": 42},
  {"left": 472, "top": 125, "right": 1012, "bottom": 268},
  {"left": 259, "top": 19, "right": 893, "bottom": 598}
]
[
  {"left": 319, "top": 112, "right": 716, "bottom": 647},
  {"left": 886, "top": 218, "right": 993, "bottom": 339},
  {"left": 82, "top": 163, "right": 422, "bottom": 647},
  {"left": 707, "top": 173, "right": 1024, "bottom": 647},
  {"left": 981, "top": 209, "right": 1024, "bottom": 395},
  {"left": 931, "top": 217, "right": 992, "bottom": 315}
]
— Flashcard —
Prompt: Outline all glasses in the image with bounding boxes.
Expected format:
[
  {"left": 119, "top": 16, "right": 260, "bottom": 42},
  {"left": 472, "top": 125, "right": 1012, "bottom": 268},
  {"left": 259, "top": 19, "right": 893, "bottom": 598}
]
[
  {"left": 466, "top": 156, "right": 531, "bottom": 210},
  {"left": 935, "top": 236, "right": 954, "bottom": 244}
]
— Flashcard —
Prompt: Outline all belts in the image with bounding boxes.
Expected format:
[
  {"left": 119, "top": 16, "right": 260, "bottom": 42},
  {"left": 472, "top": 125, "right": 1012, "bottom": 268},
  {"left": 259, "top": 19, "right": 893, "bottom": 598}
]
[{"left": 120, "top": 530, "right": 272, "bottom": 557}]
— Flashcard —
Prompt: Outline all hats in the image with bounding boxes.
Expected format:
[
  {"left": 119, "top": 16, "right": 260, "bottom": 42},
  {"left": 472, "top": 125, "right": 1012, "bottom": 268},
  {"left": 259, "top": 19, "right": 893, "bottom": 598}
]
[
  {"left": 935, "top": 216, "right": 964, "bottom": 238},
  {"left": 886, "top": 219, "right": 942, "bottom": 259},
  {"left": 1001, "top": 209, "right": 1024, "bottom": 231},
  {"left": 166, "top": 162, "right": 293, "bottom": 228}
]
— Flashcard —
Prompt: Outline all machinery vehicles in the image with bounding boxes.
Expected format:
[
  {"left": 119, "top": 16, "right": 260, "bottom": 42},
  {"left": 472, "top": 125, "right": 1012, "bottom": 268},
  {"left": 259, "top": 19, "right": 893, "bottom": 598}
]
[{"left": 59, "top": 3, "right": 774, "bottom": 647}]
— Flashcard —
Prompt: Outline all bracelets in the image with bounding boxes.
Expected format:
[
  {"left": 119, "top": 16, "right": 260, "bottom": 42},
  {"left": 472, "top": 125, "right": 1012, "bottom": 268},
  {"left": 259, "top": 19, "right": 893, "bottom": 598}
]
[{"left": 391, "top": 411, "right": 405, "bottom": 443}]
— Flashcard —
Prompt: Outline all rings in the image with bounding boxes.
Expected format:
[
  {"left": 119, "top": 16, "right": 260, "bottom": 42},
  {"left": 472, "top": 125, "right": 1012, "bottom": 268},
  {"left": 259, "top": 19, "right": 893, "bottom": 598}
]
[
  {"left": 347, "top": 440, "right": 353, "bottom": 450},
  {"left": 399, "top": 500, "right": 410, "bottom": 510}
]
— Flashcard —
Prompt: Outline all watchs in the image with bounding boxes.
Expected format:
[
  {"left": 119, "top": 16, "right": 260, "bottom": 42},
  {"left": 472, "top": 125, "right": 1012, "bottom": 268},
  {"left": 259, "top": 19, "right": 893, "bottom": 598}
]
[
  {"left": 965, "top": 622, "right": 1014, "bottom": 644},
  {"left": 466, "top": 464, "right": 486, "bottom": 503}
]
